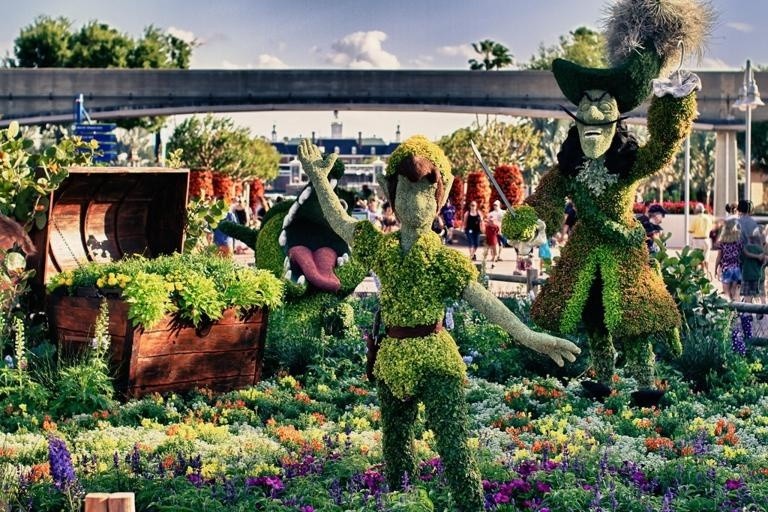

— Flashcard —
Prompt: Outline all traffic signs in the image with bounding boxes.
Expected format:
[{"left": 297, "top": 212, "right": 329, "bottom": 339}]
[
  {"left": 73, "top": 152, "right": 117, "bottom": 163},
  {"left": 73, "top": 142, "right": 119, "bottom": 152},
  {"left": 73, "top": 133, "right": 118, "bottom": 142},
  {"left": 74, "top": 123, "right": 116, "bottom": 131}
]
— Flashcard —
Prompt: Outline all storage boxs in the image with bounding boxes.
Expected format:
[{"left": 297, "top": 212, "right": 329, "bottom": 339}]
[{"left": 17, "top": 164, "right": 271, "bottom": 405}]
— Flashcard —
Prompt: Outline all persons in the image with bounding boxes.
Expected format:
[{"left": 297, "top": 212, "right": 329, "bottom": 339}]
[
  {"left": 295, "top": 134, "right": 584, "bottom": 511},
  {"left": 198, "top": 184, "right": 766, "bottom": 302}
]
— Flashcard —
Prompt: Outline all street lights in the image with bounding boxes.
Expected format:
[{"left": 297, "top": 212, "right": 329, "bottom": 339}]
[{"left": 733, "top": 57, "right": 767, "bottom": 207}]
